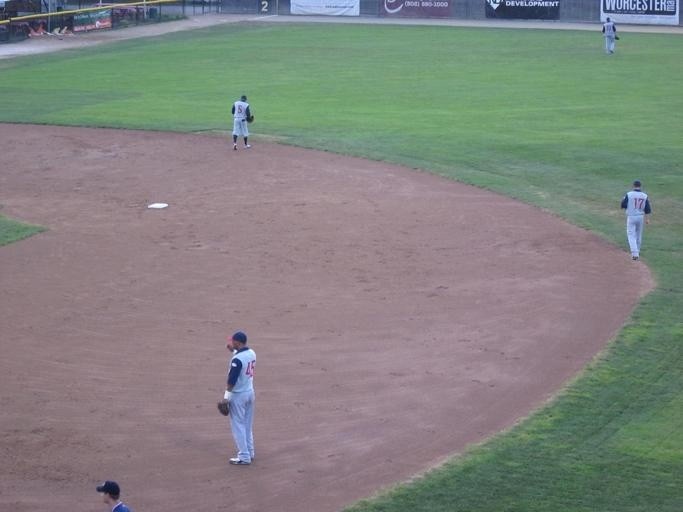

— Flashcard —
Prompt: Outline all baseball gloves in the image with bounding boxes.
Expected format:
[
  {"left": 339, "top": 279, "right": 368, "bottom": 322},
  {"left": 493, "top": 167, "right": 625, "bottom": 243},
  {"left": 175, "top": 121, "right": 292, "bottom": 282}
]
[
  {"left": 245, "top": 116, "right": 254, "bottom": 122},
  {"left": 217, "top": 400, "right": 229, "bottom": 416},
  {"left": 615, "top": 34, "right": 619, "bottom": 39}
]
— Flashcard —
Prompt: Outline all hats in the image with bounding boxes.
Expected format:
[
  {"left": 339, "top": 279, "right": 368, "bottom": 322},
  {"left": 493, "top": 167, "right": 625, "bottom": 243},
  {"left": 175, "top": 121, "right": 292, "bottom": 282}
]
[
  {"left": 96, "top": 480, "right": 120, "bottom": 496},
  {"left": 226, "top": 332, "right": 248, "bottom": 343}
]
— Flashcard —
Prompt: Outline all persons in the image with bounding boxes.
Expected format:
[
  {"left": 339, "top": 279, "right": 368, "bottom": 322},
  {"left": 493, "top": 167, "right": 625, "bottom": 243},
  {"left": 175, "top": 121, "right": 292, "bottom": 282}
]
[
  {"left": 217, "top": 331, "right": 256, "bottom": 465},
  {"left": 602, "top": 17, "right": 616, "bottom": 54},
  {"left": 621, "top": 181, "right": 651, "bottom": 259},
  {"left": 96, "top": 480, "right": 130, "bottom": 512},
  {"left": 232, "top": 96, "right": 254, "bottom": 149}
]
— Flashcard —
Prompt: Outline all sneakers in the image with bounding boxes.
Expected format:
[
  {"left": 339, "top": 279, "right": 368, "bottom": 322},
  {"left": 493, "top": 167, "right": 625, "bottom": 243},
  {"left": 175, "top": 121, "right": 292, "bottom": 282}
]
[{"left": 229, "top": 457, "right": 251, "bottom": 465}]
